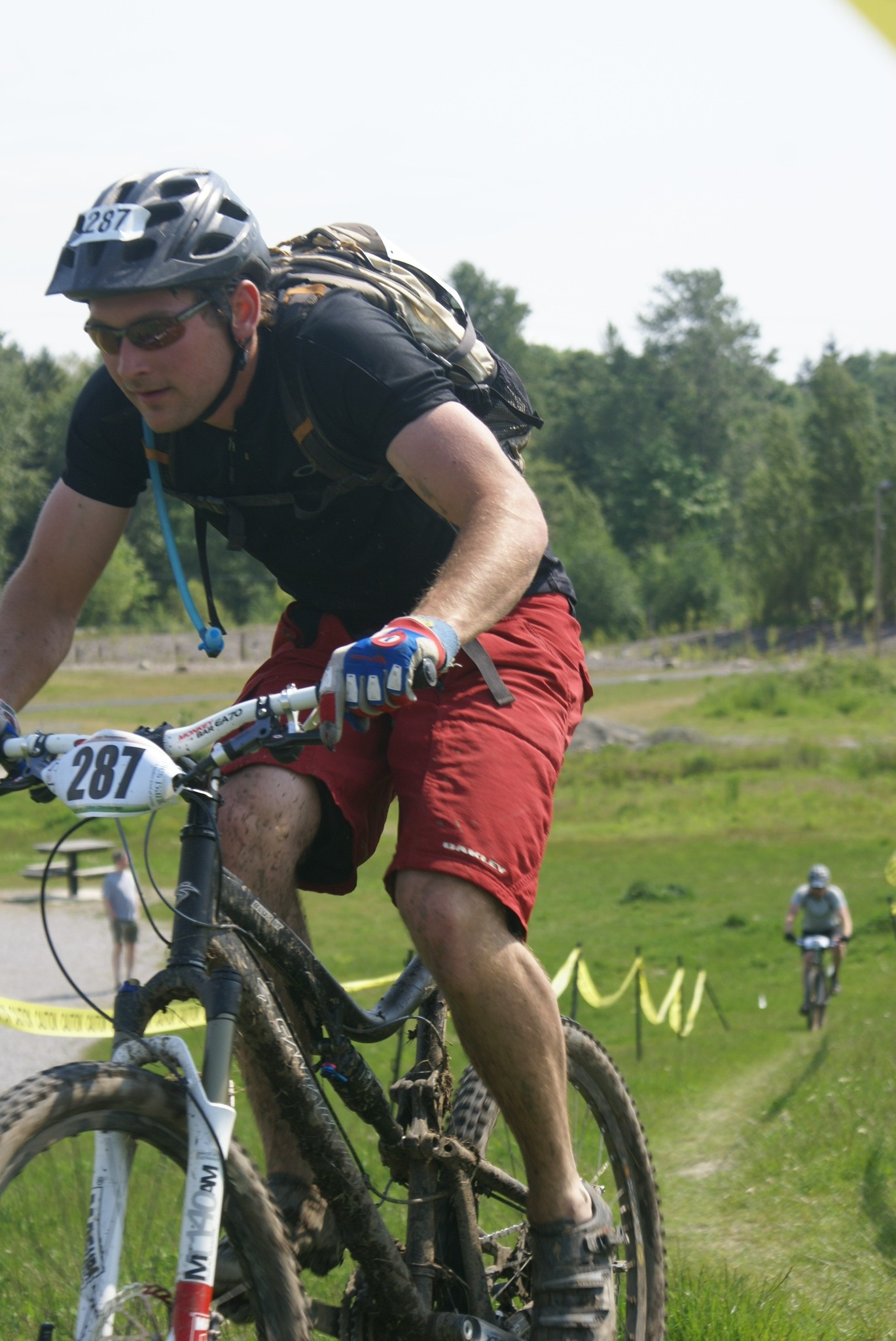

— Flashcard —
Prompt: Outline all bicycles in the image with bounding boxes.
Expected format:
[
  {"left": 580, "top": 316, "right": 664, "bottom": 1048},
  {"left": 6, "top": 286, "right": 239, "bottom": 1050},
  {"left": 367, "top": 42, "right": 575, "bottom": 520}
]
[
  {"left": 0, "top": 657, "right": 667, "bottom": 1341},
  {"left": 793, "top": 935, "right": 837, "bottom": 1030}
]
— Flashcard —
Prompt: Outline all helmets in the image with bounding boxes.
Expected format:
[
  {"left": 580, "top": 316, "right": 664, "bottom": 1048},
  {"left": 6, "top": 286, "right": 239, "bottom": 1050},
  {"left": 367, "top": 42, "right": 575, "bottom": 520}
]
[{"left": 43, "top": 168, "right": 274, "bottom": 293}]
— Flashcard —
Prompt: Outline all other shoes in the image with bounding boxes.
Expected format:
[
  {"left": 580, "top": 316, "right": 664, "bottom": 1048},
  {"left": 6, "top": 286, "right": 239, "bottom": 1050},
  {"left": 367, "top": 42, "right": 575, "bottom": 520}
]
[
  {"left": 796, "top": 1002, "right": 812, "bottom": 1016},
  {"left": 829, "top": 980, "right": 844, "bottom": 998}
]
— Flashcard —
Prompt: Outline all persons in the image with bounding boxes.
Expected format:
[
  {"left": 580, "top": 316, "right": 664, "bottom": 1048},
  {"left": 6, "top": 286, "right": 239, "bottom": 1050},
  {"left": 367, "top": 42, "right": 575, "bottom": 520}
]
[
  {"left": 0, "top": 166, "right": 617, "bottom": 1341},
  {"left": 783, "top": 864, "right": 853, "bottom": 1015}
]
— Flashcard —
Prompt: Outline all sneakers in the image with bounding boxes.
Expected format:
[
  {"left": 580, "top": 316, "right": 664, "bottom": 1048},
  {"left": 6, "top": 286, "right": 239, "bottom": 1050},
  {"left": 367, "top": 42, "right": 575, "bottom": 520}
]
[
  {"left": 215, "top": 1185, "right": 340, "bottom": 1300},
  {"left": 520, "top": 1181, "right": 627, "bottom": 1341}
]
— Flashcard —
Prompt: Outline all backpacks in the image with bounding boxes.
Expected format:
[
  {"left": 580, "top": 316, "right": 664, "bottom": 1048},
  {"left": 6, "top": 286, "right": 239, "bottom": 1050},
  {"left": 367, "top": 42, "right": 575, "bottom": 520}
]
[{"left": 264, "top": 224, "right": 546, "bottom": 489}]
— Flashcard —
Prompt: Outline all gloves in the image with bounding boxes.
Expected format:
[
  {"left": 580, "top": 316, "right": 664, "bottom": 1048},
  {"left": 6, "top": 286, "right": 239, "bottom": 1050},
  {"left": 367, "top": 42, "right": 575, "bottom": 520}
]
[
  {"left": 0, "top": 698, "right": 18, "bottom": 754},
  {"left": 319, "top": 616, "right": 461, "bottom": 745}
]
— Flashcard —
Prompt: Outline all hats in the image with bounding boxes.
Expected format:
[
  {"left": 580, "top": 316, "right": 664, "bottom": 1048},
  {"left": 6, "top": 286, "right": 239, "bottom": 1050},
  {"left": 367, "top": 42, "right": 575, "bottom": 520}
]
[{"left": 809, "top": 863, "right": 831, "bottom": 890}]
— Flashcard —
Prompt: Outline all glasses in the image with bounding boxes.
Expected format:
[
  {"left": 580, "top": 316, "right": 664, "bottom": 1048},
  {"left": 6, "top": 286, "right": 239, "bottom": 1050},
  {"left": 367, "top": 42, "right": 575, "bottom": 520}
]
[{"left": 76, "top": 294, "right": 214, "bottom": 353}]
